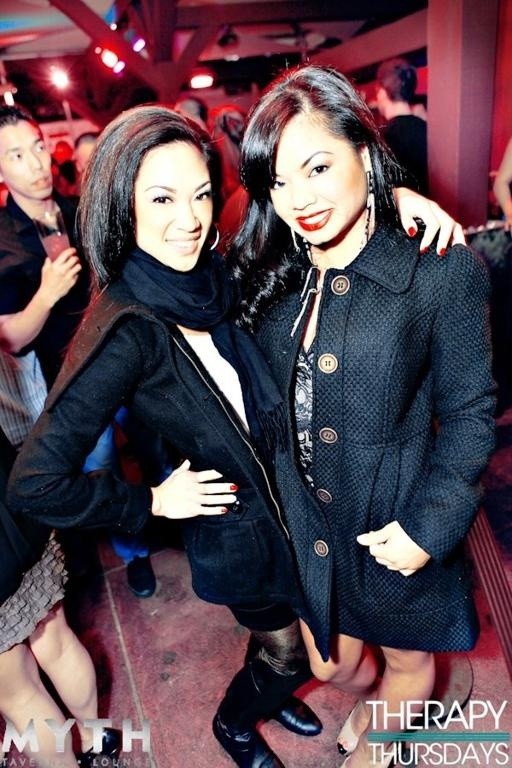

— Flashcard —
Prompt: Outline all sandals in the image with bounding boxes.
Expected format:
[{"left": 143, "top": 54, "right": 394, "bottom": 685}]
[
  {"left": 341, "top": 736, "right": 411, "bottom": 768},
  {"left": 336, "top": 698, "right": 369, "bottom": 754}
]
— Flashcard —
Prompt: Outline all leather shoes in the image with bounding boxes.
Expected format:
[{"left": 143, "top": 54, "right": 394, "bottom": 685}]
[
  {"left": 271, "top": 695, "right": 324, "bottom": 737},
  {"left": 209, "top": 710, "right": 287, "bottom": 768},
  {"left": 123, "top": 554, "right": 156, "bottom": 599}
]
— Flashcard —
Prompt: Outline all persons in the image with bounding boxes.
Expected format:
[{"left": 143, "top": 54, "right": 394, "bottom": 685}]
[
  {"left": 223, "top": 66, "right": 497, "bottom": 767},
  {"left": 0, "top": 425, "right": 123, "bottom": 768},
  {"left": 0, "top": 106, "right": 196, "bottom": 595},
  {"left": 19, "top": 56, "right": 512, "bottom": 259},
  {"left": 4, "top": 106, "right": 469, "bottom": 767}
]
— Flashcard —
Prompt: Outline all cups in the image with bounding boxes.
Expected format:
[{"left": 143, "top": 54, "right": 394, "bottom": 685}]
[{"left": 32, "top": 209, "right": 71, "bottom": 263}]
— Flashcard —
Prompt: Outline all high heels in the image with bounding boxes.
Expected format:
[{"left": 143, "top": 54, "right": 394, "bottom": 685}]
[{"left": 74, "top": 724, "right": 127, "bottom": 768}]
